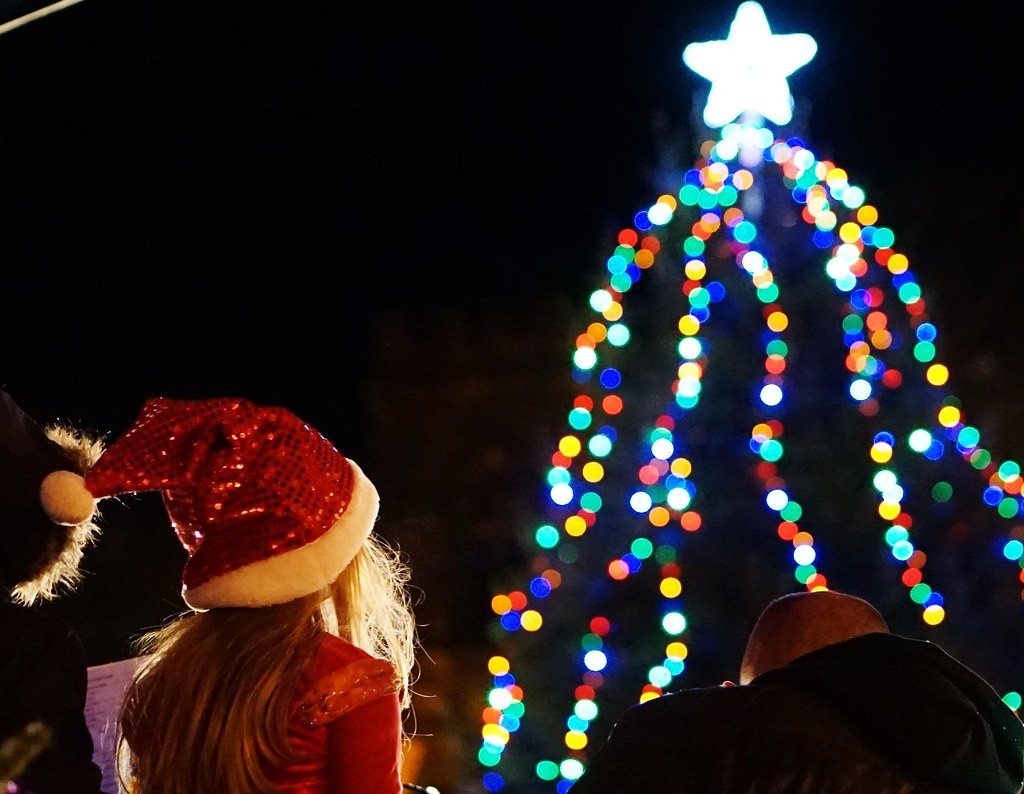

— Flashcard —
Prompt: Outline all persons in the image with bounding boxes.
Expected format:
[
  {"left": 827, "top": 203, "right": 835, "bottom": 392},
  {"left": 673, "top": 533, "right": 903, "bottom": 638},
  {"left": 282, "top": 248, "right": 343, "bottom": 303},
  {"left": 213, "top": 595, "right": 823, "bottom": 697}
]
[
  {"left": 0, "top": 378, "right": 109, "bottom": 794},
  {"left": 39, "top": 397, "right": 440, "bottom": 794},
  {"left": 568, "top": 589, "right": 1023, "bottom": 794}
]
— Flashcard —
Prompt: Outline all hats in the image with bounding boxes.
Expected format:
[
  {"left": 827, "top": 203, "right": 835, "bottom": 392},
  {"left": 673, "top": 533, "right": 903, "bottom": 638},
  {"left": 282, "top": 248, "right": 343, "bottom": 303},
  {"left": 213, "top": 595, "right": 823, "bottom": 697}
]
[{"left": 42, "top": 397, "right": 381, "bottom": 610}]
